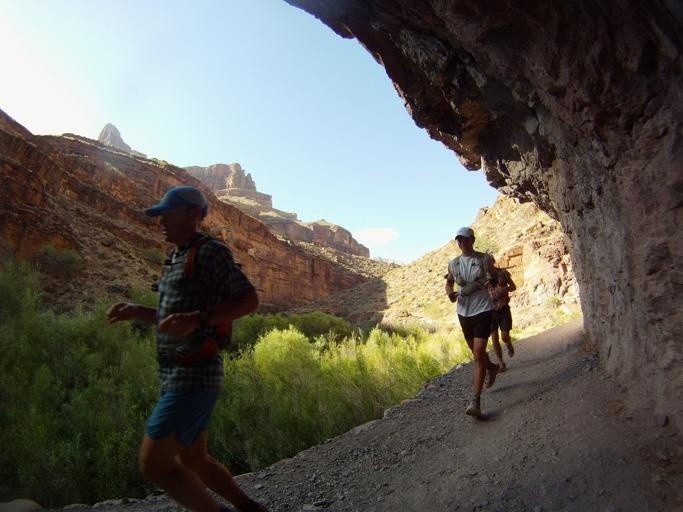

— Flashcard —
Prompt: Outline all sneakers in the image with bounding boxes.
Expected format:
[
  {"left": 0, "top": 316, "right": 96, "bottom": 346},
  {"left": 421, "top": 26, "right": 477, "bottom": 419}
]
[
  {"left": 497, "top": 362, "right": 506, "bottom": 372},
  {"left": 507, "top": 344, "right": 513, "bottom": 357},
  {"left": 464, "top": 401, "right": 481, "bottom": 416},
  {"left": 485, "top": 363, "right": 499, "bottom": 388}
]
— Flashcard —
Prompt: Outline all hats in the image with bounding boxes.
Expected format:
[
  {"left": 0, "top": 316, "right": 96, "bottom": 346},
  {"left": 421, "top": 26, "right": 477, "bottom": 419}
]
[
  {"left": 144, "top": 186, "right": 208, "bottom": 218},
  {"left": 454, "top": 226, "right": 474, "bottom": 238}
]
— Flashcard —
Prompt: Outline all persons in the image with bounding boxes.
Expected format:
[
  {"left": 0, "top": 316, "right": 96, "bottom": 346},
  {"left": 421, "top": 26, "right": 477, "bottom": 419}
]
[
  {"left": 106, "top": 186, "right": 270, "bottom": 512},
  {"left": 486, "top": 254, "right": 516, "bottom": 373},
  {"left": 445, "top": 227, "right": 500, "bottom": 418}
]
